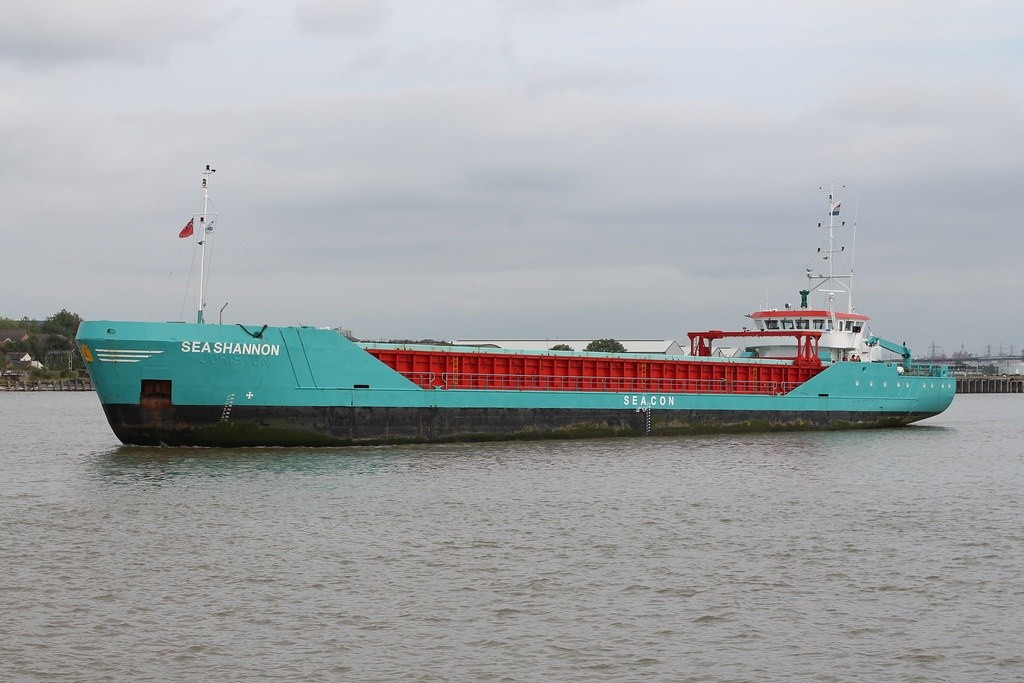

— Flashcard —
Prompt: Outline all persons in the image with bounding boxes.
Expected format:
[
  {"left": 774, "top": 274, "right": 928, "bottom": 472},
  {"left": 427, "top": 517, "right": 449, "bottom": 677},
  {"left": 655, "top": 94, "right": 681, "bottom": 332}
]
[
  {"left": 856, "top": 328, "right": 860, "bottom": 333},
  {"left": 850, "top": 354, "right": 860, "bottom": 362},
  {"left": 897, "top": 362, "right": 904, "bottom": 375},
  {"left": 843, "top": 355, "right": 848, "bottom": 361}
]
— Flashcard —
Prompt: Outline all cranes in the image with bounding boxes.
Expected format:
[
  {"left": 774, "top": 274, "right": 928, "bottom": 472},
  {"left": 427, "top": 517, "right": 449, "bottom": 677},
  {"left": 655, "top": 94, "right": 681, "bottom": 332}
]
[{"left": 927, "top": 341, "right": 1016, "bottom": 359}]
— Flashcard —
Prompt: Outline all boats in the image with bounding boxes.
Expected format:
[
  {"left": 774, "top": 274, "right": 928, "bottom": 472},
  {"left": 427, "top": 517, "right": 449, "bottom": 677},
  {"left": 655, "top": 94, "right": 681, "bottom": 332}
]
[
  {"left": 990, "top": 361, "right": 999, "bottom": 366},
  {"left": 74, "top": 163, "right": 957, "bottom": 448}
]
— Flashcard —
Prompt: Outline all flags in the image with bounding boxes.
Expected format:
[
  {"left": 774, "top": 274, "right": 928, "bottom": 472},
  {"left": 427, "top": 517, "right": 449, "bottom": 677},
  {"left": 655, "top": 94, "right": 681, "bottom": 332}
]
[{"left": 178, "top": 217, "right": 194, "bottom": 238}]
